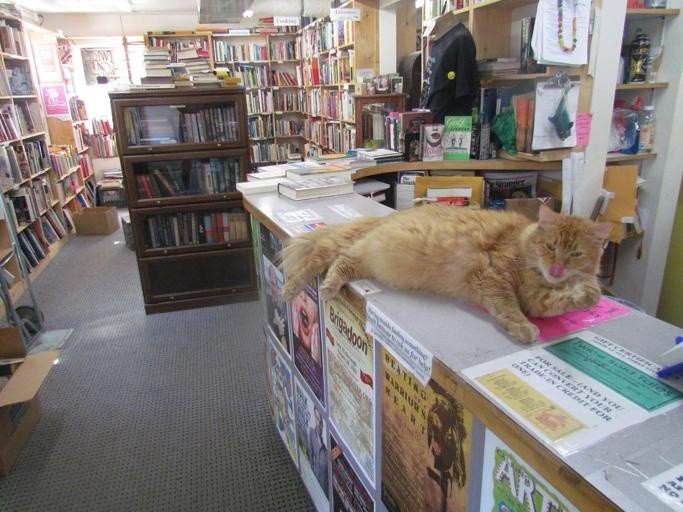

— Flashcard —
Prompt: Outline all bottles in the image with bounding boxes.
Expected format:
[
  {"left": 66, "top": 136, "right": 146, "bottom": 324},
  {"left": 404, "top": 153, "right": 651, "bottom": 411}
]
[
  {"left": 629, "top": 34, "right": 650, "bottom": 82},
  {"left": 622, "top": 105, "right": 657, "bottom": 154}
]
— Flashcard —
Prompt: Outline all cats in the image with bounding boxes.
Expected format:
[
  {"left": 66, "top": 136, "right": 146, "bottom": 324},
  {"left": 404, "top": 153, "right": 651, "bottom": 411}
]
[{"left": 271, "top": 204, "right": 615, "bottom": 344}]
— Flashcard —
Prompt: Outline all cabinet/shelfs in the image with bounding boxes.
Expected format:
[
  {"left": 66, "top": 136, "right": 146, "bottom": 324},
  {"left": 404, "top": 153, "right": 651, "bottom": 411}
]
[{"left": 0, "top": 0, "right": 683, "bottom": 315}]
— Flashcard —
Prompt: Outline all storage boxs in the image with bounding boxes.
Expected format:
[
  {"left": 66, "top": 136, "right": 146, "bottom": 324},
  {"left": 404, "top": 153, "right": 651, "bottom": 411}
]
[{"left": 0, "top": 327, "right": 63, "bottom": 477}]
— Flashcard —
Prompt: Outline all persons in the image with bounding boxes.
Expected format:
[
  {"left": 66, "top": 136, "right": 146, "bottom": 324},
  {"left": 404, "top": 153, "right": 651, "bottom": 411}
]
[
  {"left": 292, "top": 290, "right": 322, "bottom": 366},
  {"left": 419, "top": 13, "right": 477, "bottom": 123},
  {"left": 266, "top": 264, "right": 278, "bottom": 298}
]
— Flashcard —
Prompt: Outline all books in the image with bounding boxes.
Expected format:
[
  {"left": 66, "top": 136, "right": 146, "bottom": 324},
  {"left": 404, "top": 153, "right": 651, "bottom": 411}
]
[
  {"left": 237, "top": 148, "right": 404, "bottom": 199},
  {"left": 304, "top": 10, "right": 356, "bottom": 158},
  {"left": 92, "top": 119, "right": 119, "bottom": 157},
  {"left": 1, "top": 1, "right": 68, "bottom": 288},
  {"left": 121, "top": 102, "right": 237, "bottom": 147},
  {"left": 477, "top": 57, "right": 521, "bottom": 76},
  {"left": 134, "top": 160, "right": 242, "bottom": 200},
  {"left": 521, "top": 16, "right": 545, "bottom": 73},
  {"left": 145, "top": 209, "right": 253, "bottom": 251},
  {"left": 438, "top": 0, "right": 467, "bottom": 17},
  {"left": 60, "top": 39, "right": 101, "bottom": 205},
  {"left": 214, "top": 38, "right": 277, "bottom": 169},
  {"left": 270, "top": 40, "right": 305, "bottom": 164},
  {"left": 358, "top": 74, "right": 546, "bottom": 211},
  {"left": 132, "top": 38, "right": 241, "bottom": 89},
  {"left": 48, "top": 145, "right": 89, "bottom": 217}
]
